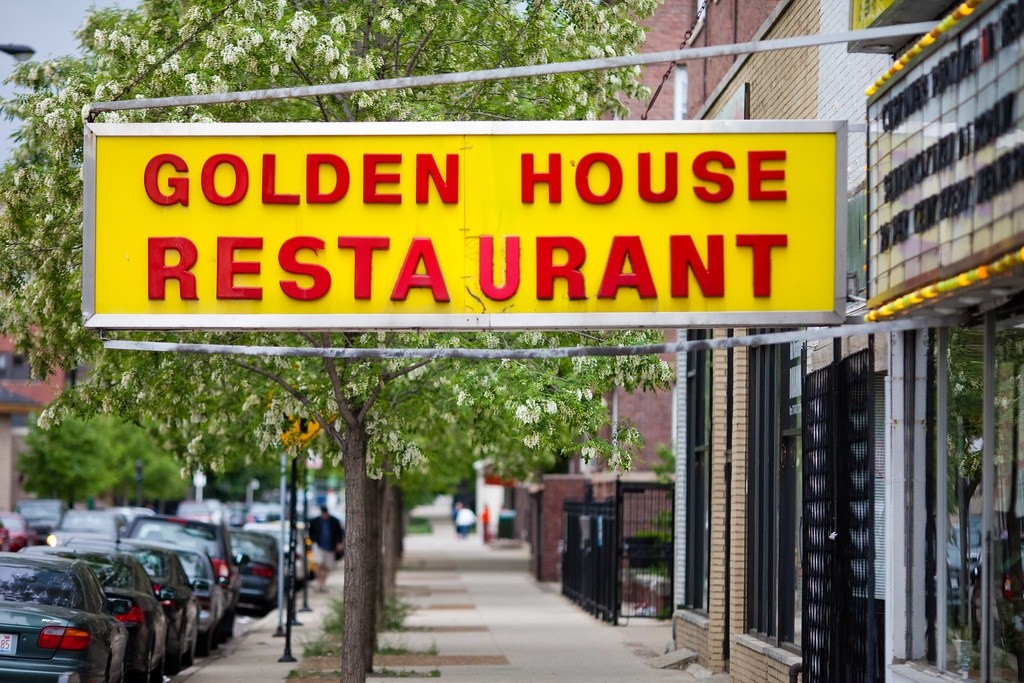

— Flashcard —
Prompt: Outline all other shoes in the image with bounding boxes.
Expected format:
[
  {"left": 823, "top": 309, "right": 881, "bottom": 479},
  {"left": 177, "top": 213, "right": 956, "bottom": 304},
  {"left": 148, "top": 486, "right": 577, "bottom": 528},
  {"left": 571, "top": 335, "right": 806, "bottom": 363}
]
[{"left": 315, "top": 587, "right": 328, "bottom": 593}]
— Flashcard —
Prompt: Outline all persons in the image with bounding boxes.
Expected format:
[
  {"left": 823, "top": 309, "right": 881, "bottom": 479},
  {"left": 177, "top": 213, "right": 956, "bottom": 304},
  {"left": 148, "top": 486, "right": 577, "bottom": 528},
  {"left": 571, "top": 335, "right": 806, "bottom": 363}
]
[
  {"left": 452, "top": 501, "right": 476, "bottom": 540},
  {"left": 309, "top": 506, "right": 345, "bottom": 592}
]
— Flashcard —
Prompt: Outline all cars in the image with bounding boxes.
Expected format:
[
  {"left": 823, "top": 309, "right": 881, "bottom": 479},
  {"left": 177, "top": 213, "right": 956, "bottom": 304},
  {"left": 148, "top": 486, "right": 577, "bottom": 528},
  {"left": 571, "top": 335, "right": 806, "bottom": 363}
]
[
  {"left": 57, "top": 537, "right": 211, "bottom": 678},
  {"left": 13, "top": 498, "right": 69, "bottom": 546},
  {"left": 0, "top": 552, "right": 133, "bottom": 683},
  {"left": 127, "top": 513, "right": 251, "bottom": 644},
  {"left": 229, "top": 529, "right": 299, "bottom": 617},
  {"left": 48, "top": 511, "right": 130, "bottom": 551},
  {"left": 947, "top": 515, "right": 981, "bottom": 626},
  {"left": 970, "top": 536, "right": 1024, "bottom": 654},
  {"left": 175, "top": 501, "right": 279, "bottom": 527},
  {"left": 122, "top": 538, "right": 230, "bottom": 657},
  {"left": 17, "top": 546, "right": 177, "bottom": 683},
  {"left": 245, "top": 521, "right": 303, "bottom": 591},
  {"left": 0, "top": 512, "right": 39, "bottom": 553}
]
